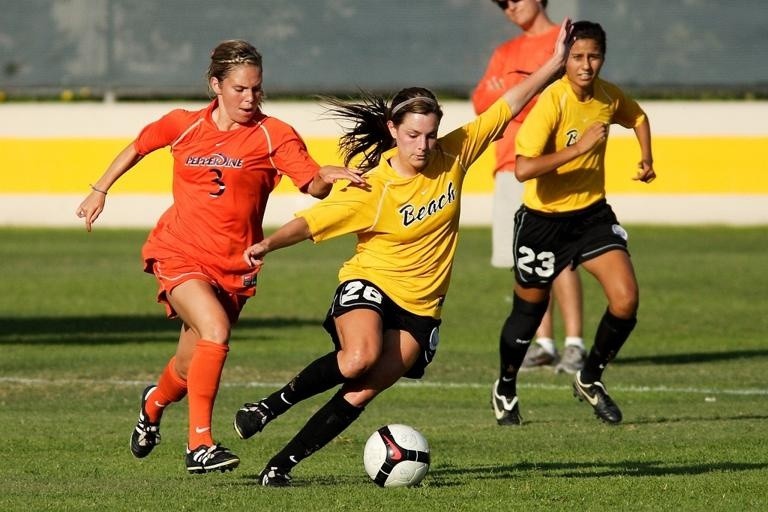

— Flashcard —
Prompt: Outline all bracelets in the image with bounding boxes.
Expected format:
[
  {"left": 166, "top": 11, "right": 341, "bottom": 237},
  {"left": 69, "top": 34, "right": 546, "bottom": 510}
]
[{"left": 89, "top": 182, "right": 110, "bottom": 197}]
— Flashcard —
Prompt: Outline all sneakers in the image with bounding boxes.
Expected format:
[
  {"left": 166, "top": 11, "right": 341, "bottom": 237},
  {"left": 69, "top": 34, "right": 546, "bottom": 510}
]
[
  {"left": 232, "top": 399, "right": 272, "bottom": 439},
  {"left": 185, "top": 441, "right": 241, "bottom": 474},
  {"left": 129, "top": 385, "right": 162, "bottom": 459},
  {"left": 490, "top": 378, "right": 522, "bottom": 425},
  {"left": 522, "top": 340, "right": 554, "bottom": 367},
  {"left": 258, "top": 465, "right": 293, "bottom": 487},
  {"left": 572, "top": 370, "right": 623, "bottom": 423},
  {"left": 555, "top": 345, "right": 587, "bottom": 376}
]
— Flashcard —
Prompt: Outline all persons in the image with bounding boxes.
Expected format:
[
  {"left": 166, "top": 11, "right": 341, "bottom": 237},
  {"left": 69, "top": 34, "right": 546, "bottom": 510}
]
[
  {"left": 489, "top": 17, "right": 656, "bottom": 426},
  {"left": 233, "top": 14, "right": 573, "bottom": 488},
  {"left": 74, "top": 35, "right": 368, "bottom": 475},
  {"left": 470, "top": 0, "right": 591, "bottom": 377}
]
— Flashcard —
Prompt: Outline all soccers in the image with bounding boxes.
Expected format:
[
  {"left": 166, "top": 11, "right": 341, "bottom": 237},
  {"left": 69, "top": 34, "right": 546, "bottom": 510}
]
[{"left": 364, "top": 424, "right": 430, "bottom": 488}]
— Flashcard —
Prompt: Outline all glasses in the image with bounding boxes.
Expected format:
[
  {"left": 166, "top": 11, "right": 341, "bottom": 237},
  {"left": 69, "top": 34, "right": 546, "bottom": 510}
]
[{"left": 496, "top": 0, "right": 522, "bottom": 10}]
